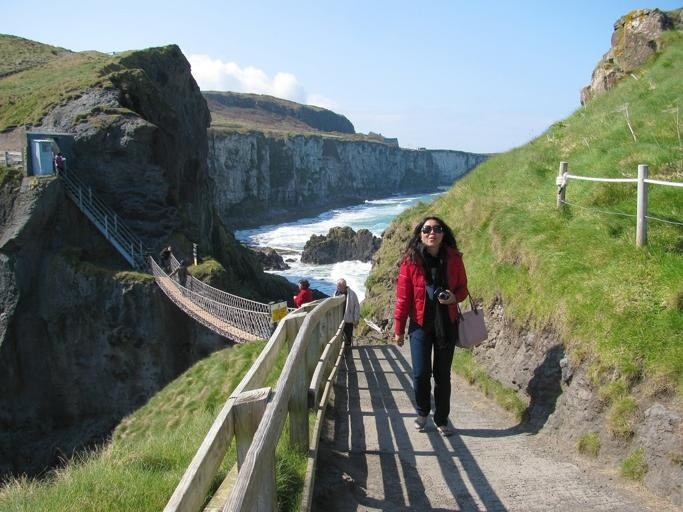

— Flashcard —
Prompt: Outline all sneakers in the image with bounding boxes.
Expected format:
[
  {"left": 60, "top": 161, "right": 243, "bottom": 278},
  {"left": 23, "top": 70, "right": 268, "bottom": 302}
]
[
  {"left": 414, "top": 417, "right": 427, "bottom": 429},
  {"left": 436, "top": 426, "right": 452, "bottom": 436}
]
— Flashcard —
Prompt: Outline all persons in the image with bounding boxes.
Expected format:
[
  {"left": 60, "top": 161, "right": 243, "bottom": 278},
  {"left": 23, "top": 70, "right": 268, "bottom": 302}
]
[
  {"left": 333, "top": 279, "right": 361, "bottom": 348},
  {"left": 168, "top": 259, "right": 190, "bottom": 290},
  {"left": 54, "top": 152, "right": 67, "bottom": 176},
  {"left": 292, "top": 279, "right": 314, "bottom": 307},
  {"left": 158, "top": 248, "right": 172, "bottom": 272},
  {"left": 395, "top": 217, "right": 468, "bottom": 436}
]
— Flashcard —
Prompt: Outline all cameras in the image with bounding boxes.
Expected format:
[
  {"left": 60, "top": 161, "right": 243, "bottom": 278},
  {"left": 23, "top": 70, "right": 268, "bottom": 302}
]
[{"left": 434, "top": 288, "right": 449, "bottom": 300}]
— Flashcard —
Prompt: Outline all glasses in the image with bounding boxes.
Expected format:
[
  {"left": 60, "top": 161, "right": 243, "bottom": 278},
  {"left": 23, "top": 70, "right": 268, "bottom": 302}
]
[{"left": 421, "top": 225, "right": 444, "bottom": 234}]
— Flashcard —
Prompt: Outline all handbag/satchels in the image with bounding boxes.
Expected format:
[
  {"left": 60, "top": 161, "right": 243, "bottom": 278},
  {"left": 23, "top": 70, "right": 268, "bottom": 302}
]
[{"left": 456, "top": 286, "right": 488, "bottom": 348}]
